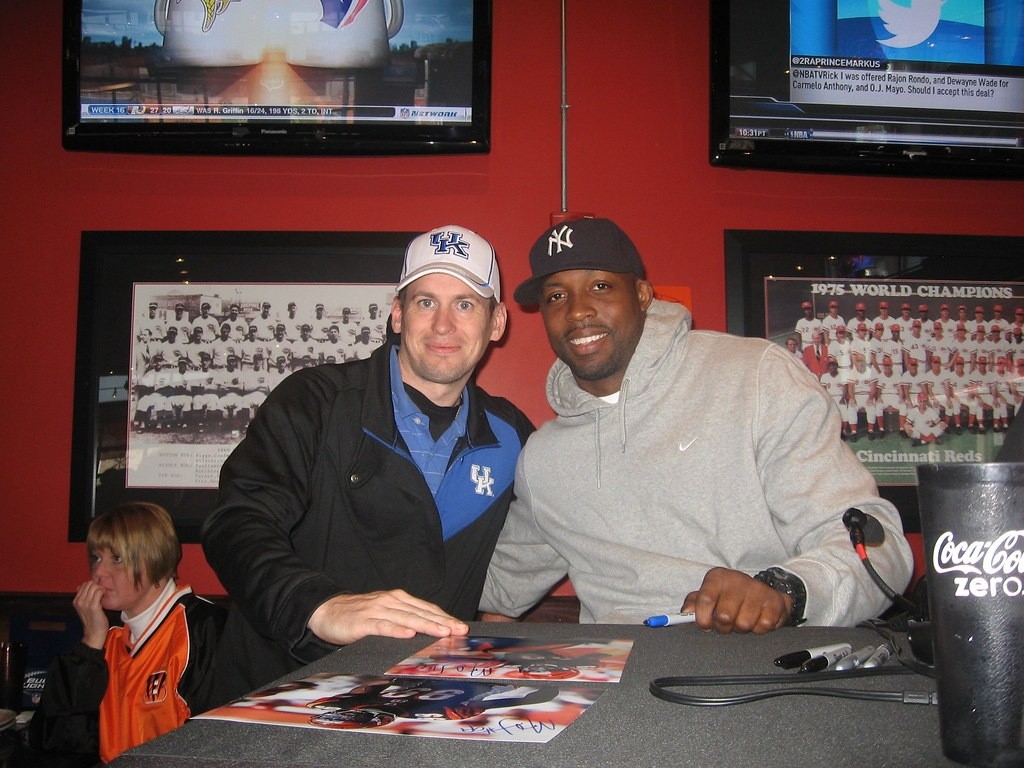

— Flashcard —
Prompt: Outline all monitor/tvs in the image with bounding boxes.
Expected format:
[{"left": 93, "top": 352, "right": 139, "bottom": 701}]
[
  {"left": 61, "top": 0, "right": 494, "bottom": 159},
  {"left": 708, "top": 0, "right": 1024, "bottom": 179}
]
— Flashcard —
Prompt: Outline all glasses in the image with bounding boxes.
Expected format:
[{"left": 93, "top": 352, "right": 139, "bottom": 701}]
[{"left": 919, "top": 399, "right": 927, "bottom": 403}]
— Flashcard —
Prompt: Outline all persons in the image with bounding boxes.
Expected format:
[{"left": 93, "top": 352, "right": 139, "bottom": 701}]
[
  {"left": 477, "top": 217, "right": 915, "bottom": 636},
  {"left": 303, "top": 623, "right": 607, "bottom": 729},
  {"left": 133, "top": 302, "right": 385, "bottom": 433},
  {"left": 199, "top": 224, "right": 536, "bottom": 707},
  {"left": 785, "top": 300, "right": 1024, "bottom": 448},
  {"left": 25, "top": 502, "right": 225, "bottom": 768}
]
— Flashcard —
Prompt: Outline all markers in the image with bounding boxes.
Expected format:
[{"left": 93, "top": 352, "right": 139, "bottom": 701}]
[
  {"left": 798, "top": 647, "right": 852, "bottom": 673},
  {"left": 774, "top": 643, "right": 852, "bottom": 670},
  {"left": 836, "top": 645, "right": 875, "bottom": 671},
  {"left": 863, "top": 643, "right": 893, "bottom": 668},
  {"left": 643, "top": 613, "right": 695, "bottom": 627}
]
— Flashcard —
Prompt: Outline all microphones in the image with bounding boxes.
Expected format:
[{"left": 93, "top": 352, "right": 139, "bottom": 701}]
[{"left": 843, "top": 507, "right": 886, "bottom": 547}]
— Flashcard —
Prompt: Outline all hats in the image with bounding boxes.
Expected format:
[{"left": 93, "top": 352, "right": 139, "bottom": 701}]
[
  {"left": 513, "top": 218, "right": 645, "bottom": 305},
  {"left": 826, "top": 354, "right": 1024, "bottom": 370},
  {"left": 145, "top": 300, "right": 379, "bottom": 369},
  {"left": 918, "top": 393, "right": 928, "bottom": 400},
  {"left": 835, "top": 322, "right": 1022, "bottom": 337},
  {"left": 395, "top": 224, "right": 500, "bottom": 304},
  {"left": 801, "top": 301, "right": 1024, "bottom": 315}
]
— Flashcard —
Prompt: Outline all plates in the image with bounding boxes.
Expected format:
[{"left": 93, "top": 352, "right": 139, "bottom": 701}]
[{"left": 0, "top": 709, "right": 16, "bottom": 732}]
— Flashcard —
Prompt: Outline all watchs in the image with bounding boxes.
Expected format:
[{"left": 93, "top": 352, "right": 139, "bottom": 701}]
[{"left": 753, "top": 567, "right": 807, "bottom": 628}]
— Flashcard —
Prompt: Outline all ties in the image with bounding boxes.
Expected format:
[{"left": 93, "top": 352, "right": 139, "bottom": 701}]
[{"left": 816, "top": 346, "right": 821, "bottom": 361}]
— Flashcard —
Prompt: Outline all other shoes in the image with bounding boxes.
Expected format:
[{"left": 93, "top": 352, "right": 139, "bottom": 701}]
[
  {"left": 955, "top": 426, "right": 962, "bottom": 435},
  {"left": 993, "top": 426, "right": 1001, "bottom": 432},
  {"left": 1004, "top": 427, "right": 1009, "bottom": 432},
  {"left": 132, "top": 407, "right": 251, "bottom": 436},
  {"left": 868, "top": 432, "right": 875, "bottom": 440},
  {"left": 841, "top": 433, "right": 848, "bottom": 441},
  {"left": 900, "top": 430, "right": 908, "bottom": 438},
  {"left": 879, "top": 430, "right": 885, "bottom": 439},
  {"left": 945, "top": 426, "right": 951, "bottom": 434},
  {"left": 850, "top": 433, "right": 857, "bottom": 443},
  {"left": 979, "top": 428, "right": 985, "bottom": 433},
  {"left": 912, "top": 438, "right": 926, "bottom": 447},
  {"left": 968, "top": 425, "right": 977, "bottom": 434}
]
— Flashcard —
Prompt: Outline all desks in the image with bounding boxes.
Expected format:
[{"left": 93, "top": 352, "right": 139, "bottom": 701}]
[{"left": 102, "top": 621, "right": 961, "bottom": 768}]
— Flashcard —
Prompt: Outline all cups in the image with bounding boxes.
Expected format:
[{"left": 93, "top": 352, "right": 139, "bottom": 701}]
[
  {"left": 915, "top": 458, "right": 1023, "bottom": 768},
  {"left": 0, "top": 641, "right": 26, "bottom": 715}
]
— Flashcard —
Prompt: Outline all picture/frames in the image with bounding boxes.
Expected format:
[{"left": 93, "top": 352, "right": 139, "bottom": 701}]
[
  {"left": 724, "top": 228, "right": 1024, "bottom": 535},
  {"left": 66, "top": 230, "right": 428, "bottom": 545}
]
